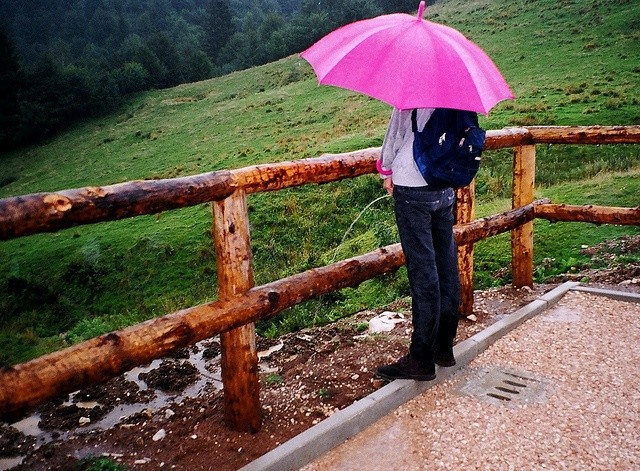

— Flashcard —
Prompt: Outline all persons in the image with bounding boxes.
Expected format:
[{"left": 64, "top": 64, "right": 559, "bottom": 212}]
[{"left": 374, "top": 106, "right": 460, "bottom": 382}]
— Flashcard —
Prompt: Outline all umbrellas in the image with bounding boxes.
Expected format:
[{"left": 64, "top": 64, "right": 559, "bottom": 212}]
[{"left": 298, "top": 0, "right": 517, "bottom": 120}]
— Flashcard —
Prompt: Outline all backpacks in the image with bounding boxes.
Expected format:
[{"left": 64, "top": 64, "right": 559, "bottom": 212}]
[{"left": 411, "top": 108, "right": 486, "bottom": 191}]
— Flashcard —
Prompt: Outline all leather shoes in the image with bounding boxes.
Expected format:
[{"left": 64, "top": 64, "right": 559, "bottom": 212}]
[
  {"left": 434, "top": 344, "right": 456, "bottom": 367},
  {"left": 376, "top": 353, "right": 436, "bottom": 380}
]
